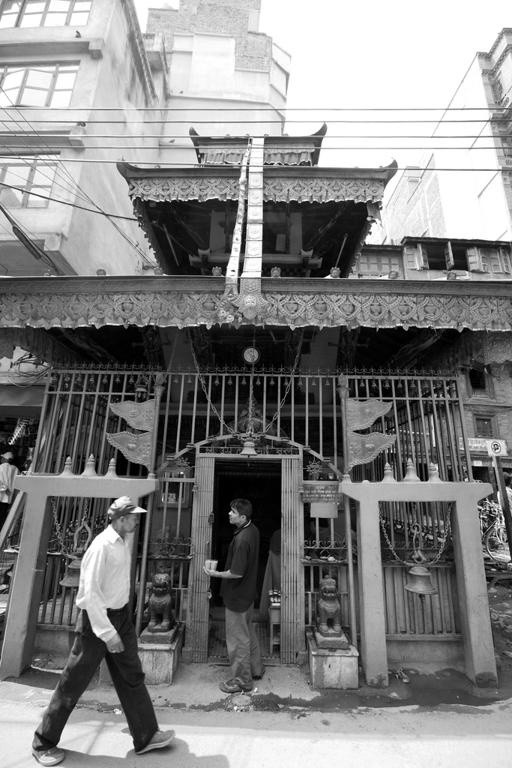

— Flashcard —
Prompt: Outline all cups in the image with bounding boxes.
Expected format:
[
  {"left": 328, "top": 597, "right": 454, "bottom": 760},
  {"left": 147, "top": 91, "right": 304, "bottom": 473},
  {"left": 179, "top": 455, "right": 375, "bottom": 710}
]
[{"left": 205, "top": 560, "right": 218, "bottom": 571}]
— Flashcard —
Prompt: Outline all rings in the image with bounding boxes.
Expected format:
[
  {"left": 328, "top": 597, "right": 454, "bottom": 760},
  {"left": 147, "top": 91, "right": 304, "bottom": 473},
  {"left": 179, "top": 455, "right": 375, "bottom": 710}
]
[{"left": 112, "top": 650, "right": 116, "bottom": 654}]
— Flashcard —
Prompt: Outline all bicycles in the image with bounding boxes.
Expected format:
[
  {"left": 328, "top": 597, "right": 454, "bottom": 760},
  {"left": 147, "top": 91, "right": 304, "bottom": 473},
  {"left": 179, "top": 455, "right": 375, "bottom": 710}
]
[{"left": 477, "top": 498, "right": 512, "bottom": 566}]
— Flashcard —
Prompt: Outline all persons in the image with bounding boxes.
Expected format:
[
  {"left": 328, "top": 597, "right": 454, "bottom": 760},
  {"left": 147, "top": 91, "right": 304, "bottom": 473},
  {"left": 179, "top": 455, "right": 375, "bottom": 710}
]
[
  {"left": 202, "top": 498, "right": 263, "bottom": 695},
  {"left": 0, "top": 452, "right": 19, "bottom": 525},
  {"left": 32, "top": 495, "right": 174, "bottom": 766}
]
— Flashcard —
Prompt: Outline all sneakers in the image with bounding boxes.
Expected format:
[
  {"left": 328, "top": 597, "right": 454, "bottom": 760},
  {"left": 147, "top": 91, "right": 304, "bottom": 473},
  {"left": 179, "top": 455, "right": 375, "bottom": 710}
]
[
  {"left": 137, "top": 730, "right": 173, "bottom": 754},
  {"left": 219, "top": 680, "right": 252, "bottom": 693},
  {"left": 33, "top": 747, "right": 64, "bottom": 766}
]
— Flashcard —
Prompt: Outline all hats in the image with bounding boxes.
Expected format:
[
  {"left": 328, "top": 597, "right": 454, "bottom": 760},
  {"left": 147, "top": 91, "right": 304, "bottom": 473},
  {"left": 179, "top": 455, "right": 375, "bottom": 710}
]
[
  {"left": 108, "top": 496, "right": 148, "bottom": 517},
  {"left": 1, "top": 451, "right": 14, "bottom": 460}
]
